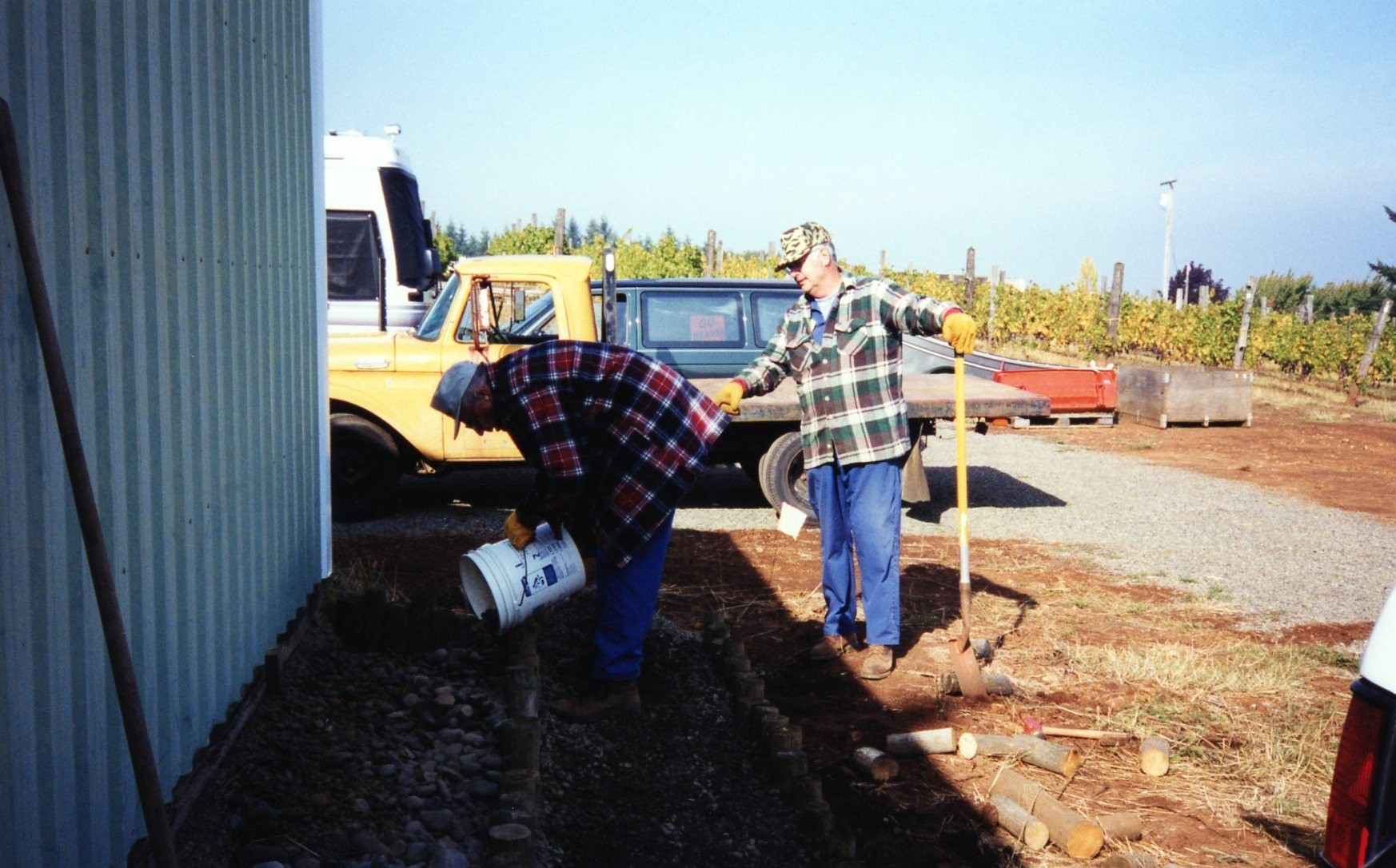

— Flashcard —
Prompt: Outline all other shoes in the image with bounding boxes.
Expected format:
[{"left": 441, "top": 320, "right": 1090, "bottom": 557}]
[
  {"left": 861, "top": 645, "right": 893, "bottom": 679},
  {"left": 544, "top": 682, "right": 642, "bottom": 723},
  {"left": 810, "top": 632, "right": 858, "bottom": 659}
]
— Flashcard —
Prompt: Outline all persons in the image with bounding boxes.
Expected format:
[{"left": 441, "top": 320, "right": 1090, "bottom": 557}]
[
  {"left": 713, "top": 223, "right": 977, "bottom": 679},
  {"left": 430, "top": 339, "right": 734, "bottom": 722}
]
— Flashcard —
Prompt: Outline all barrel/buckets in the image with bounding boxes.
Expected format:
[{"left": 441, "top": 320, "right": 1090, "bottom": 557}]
[{"left": 457, "top": 521, "right": 587, "bottom": 633}]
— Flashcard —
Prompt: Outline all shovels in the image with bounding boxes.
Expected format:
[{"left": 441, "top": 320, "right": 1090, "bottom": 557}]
[{"left": 949, "top": 343, "right": 988, "bottom": 700}]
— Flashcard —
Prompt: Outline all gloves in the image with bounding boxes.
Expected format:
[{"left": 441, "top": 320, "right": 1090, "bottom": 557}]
[
  {"left": 503, "top": 509, "right": 536, "bottom": 549},
  {"left": 712, "top": 382, "right": 743, "bottom": 415},
  {"left": 941, "top": 308, "right": 976, "bottom": 355}
]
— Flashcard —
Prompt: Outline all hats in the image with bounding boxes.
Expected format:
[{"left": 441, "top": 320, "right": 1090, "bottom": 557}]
[
  {"left": 774, "top": 221, "right": 831, "bottom": 273},
  {"left": 429, "top": 361, "right": 480, "bottom": 441}
]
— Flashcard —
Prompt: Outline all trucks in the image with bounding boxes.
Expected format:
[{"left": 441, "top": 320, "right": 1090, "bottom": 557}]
[
  {"left": 327, "top": 254, "right": 1050, "bottom": 527},
  {"left": 324, "top": 133, "right": 443, "bottom": 332},
  {"left": 492, "top": 279, "right": 807, "bottom": 380}
]
